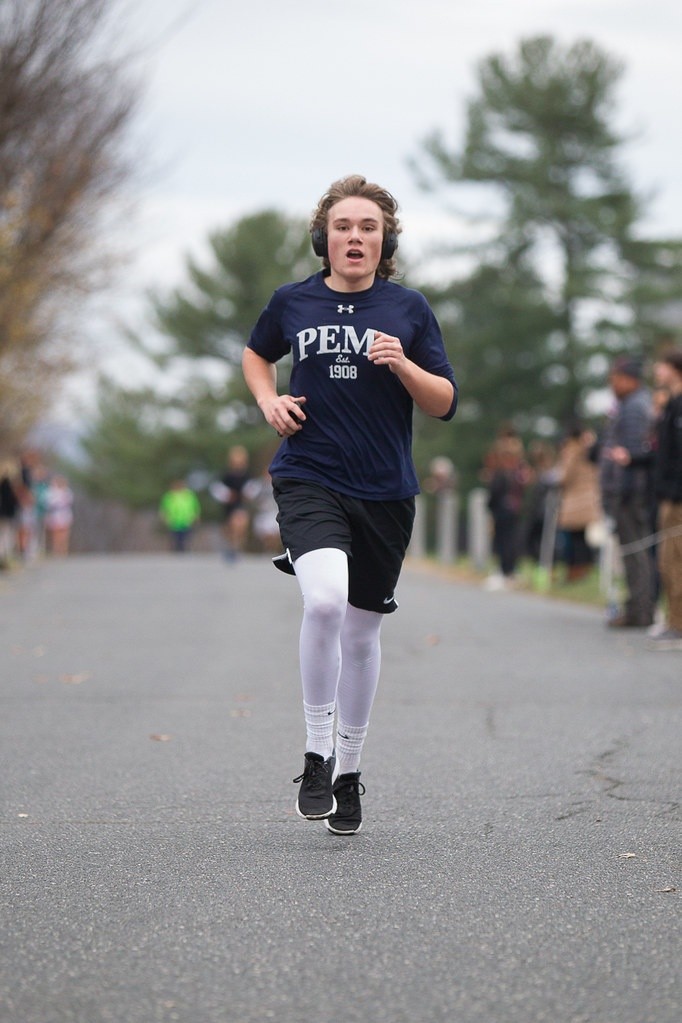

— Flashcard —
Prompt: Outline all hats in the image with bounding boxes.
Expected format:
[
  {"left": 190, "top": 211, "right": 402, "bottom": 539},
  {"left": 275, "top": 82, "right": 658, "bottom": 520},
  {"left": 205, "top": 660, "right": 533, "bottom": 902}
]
[{"left": 615, "top": 357, "right": 640, "bottom": 379}]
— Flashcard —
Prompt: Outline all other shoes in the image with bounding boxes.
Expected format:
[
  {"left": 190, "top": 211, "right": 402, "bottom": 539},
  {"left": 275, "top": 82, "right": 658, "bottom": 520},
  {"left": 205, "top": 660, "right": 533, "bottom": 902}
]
[
  {"left": 605, "top": 613, "right": 649, "bottom": 633},
  {"left": 488, "top": 568, "right": 516, "bottom": 591},
  {"left": 646, "top": 623, "right": 680, "bottom": 652}
]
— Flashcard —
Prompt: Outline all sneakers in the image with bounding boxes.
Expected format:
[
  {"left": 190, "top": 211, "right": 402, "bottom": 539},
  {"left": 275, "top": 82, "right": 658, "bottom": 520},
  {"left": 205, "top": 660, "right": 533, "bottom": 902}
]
[
  {"left": 323, "top": 766, "right": 365, "bottom": 838},
  {"left": 290, "top": 748, "right": 341, "bottom": 822}
]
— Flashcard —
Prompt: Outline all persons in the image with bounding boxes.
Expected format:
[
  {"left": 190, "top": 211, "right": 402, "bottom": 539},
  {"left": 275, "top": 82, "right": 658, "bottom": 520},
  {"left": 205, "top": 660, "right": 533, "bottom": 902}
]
[
  {"left": 433, "top": 355, "right": 682, "bottom": 652},
  {"left": 241, "top": 174, "right": 458, "bottom": 835},
  {"left": 160, "top": 446, "right": 265, "bottom": 554},
  {"left": 0, "top": 452, "right": 73, "bottom": 575}
]
